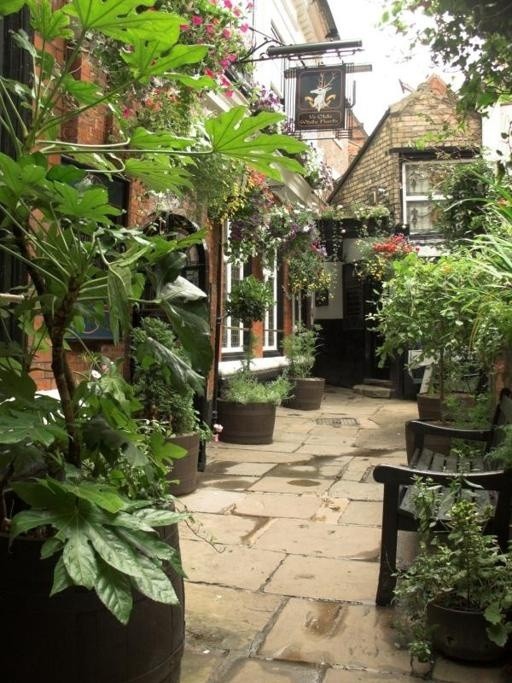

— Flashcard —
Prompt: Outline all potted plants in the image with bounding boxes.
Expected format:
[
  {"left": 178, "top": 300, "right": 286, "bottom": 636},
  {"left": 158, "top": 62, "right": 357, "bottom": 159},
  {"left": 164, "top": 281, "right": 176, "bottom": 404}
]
[
  {"left": 216, "top": 336, "right": 295, "bottom": 444},
  {"left": 283, "top": 322, "right": 326, "bottom": 411},
  {"left": 127, "top": 317, "right": 209, "bottom": 496},
  {"left": 0, "top": 1, "right": 308, "bottom": 683},
  {"left": 367, "top": 251, "right": 490, "bottom": 468},
  {"left": 394, "top": 440, "right": 512, "bottom": 668}
]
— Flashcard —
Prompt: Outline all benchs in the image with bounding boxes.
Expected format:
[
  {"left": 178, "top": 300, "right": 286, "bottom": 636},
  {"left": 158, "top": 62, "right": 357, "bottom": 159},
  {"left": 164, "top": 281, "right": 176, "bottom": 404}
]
[{"left": 374, "top": 389, "right": 512, "bottom": 607}]
